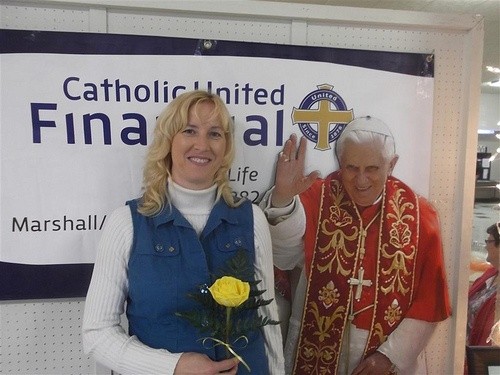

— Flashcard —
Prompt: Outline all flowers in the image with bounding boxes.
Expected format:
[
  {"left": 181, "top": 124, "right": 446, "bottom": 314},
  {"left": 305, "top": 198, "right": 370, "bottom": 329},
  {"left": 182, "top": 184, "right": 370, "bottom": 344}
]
[{"left": 170, "top": 245, "right": 283, "bottom": 369}]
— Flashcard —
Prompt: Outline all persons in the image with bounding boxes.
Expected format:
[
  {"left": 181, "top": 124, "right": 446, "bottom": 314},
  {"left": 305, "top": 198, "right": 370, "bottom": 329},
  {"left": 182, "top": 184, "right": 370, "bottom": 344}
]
[
  {"left": 82, "top": 91, "right": 286, "bottom": 375},
  {"left": 259, "top": 117, "right": 454, "bottom": 375},
  {"left": 465, "top": 223, "right": 500, "bottom": 375}
]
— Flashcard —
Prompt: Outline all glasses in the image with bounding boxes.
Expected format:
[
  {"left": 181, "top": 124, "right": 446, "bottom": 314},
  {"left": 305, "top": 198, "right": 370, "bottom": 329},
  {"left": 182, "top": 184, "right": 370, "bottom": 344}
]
[{"left": 485, "top": 240, "right": 495, "bottom": 244}]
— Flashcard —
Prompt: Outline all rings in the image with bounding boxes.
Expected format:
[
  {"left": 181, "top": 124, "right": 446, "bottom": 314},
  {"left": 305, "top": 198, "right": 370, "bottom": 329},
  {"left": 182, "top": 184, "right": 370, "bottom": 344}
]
[{"left": 284, "top": 158, "right": 289, "bottom": 162}]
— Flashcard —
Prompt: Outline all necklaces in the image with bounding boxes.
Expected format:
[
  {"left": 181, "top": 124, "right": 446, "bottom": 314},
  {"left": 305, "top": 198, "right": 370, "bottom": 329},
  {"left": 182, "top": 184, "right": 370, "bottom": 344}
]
[{"left": 347, "top": 209, "right": 382, "bottom": 303}]
